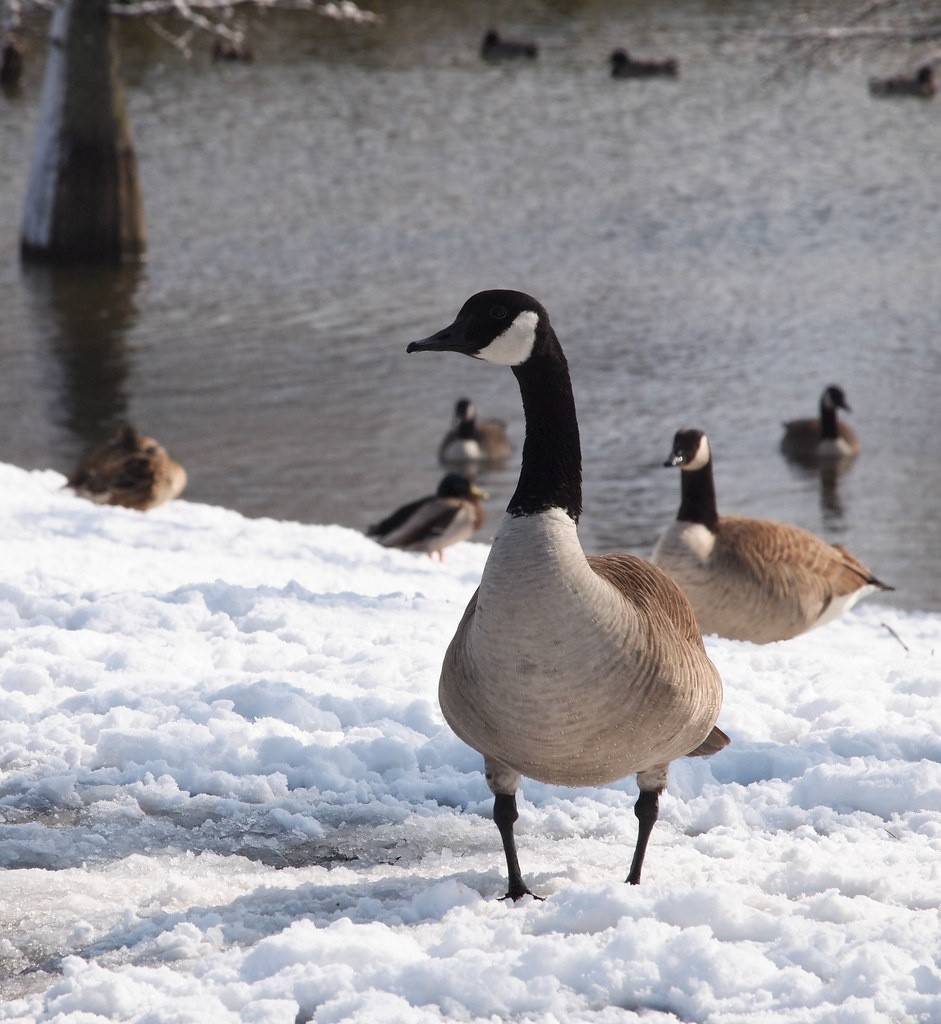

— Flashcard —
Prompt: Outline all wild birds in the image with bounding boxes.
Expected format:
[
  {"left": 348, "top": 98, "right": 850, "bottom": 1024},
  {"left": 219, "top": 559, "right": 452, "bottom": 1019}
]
[
  {"left": 408, "top": 288, "right": 731, "bottom": 904},
  {"left": 478, "top": 28, "right": 537, "bottom": 61},
  {"left": 67, "top": 422, "right": 187, "bottom": 511},
  {"left": 864, "top": 62, "right": 936, "bottom": 101},
  {"left": 436, "top": 397, "right": 516, "bottom": 463},
  {"left": 363, "top": 471, "right": 499, "bottom": 563},
  {"left": 208, "top": 33, "right": 256, "bottom": 68},
  {"left": 650, "top": 428, "right": 895, "bottom": 645},
  {"left": 780, "top": 384, "right": 860, "bottom": 460},
  {"left": 603, "top": 49, "right": 681, "bottom": 81}
]
